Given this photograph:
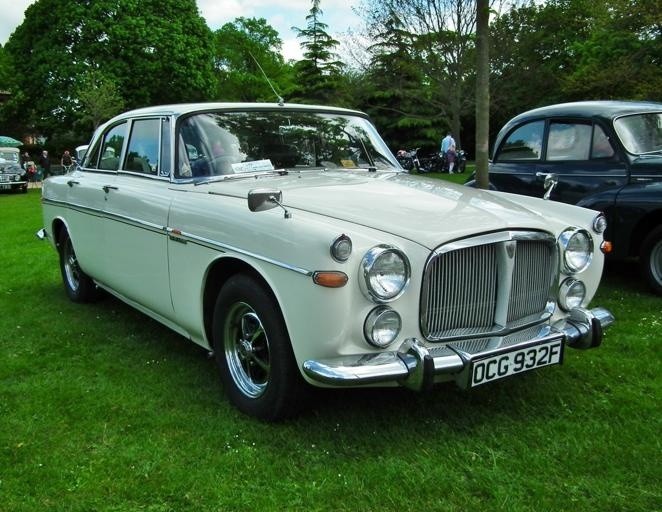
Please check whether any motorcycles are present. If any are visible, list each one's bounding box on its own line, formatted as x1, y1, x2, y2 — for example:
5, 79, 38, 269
395, 141, 467, 176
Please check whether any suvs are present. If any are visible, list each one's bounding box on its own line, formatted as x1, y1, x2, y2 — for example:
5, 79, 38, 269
0, 147, 30, 195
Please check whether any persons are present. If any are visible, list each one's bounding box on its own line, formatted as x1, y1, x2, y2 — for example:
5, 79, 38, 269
446, 144, 458, 175
60, 150, 73, 175
21, 150, 50, 180
441, 132, 456, 173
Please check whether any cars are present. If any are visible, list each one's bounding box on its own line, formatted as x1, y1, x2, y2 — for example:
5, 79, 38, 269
38, 49, 615, 423
461, 100, 662, 291
73, 144, 115, 170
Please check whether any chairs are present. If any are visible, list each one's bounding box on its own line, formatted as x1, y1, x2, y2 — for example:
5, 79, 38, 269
256, 144, 301, 169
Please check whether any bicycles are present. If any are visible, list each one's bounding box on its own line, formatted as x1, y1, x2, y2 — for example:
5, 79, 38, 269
61, 164, 74, 174
